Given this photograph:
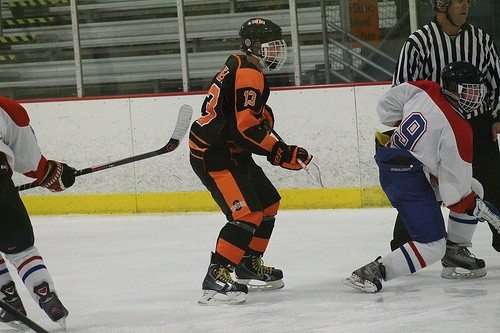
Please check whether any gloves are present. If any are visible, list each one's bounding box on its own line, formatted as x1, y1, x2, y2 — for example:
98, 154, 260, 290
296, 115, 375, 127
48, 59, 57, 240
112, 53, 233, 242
261, 105, 274, 133
467, 195, 498, 222
268, 142, 313, 171
39, 161, 76, 192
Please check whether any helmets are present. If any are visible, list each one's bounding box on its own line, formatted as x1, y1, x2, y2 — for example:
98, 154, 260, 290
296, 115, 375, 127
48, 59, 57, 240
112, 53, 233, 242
443, 62, 486, 114
240, 18, 288, 71
429, 0, 452, 11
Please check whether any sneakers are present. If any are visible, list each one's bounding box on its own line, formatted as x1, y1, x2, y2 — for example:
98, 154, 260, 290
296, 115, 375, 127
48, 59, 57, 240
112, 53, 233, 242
198, 252, 248, 305
0, 282, 29, 332
235, 255, 285, 291
441, 241, 487, 279
344, 255, 387, 292
33, 282, 70, 332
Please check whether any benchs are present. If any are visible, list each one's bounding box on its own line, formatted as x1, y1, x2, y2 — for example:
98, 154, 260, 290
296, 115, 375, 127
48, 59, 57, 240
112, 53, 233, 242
0, 0, 398, 89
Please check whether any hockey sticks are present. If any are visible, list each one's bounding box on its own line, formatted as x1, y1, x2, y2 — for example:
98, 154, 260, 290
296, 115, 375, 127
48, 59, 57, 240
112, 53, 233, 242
260, 120, 322, 187
16, 104, 193, 192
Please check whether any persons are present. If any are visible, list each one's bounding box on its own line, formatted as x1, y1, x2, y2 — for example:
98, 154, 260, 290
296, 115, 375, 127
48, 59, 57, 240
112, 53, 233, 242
353, 60, 488, 292
188, 17, 314, 294
390, 0, 500, 251
0, 95, 77, 322
